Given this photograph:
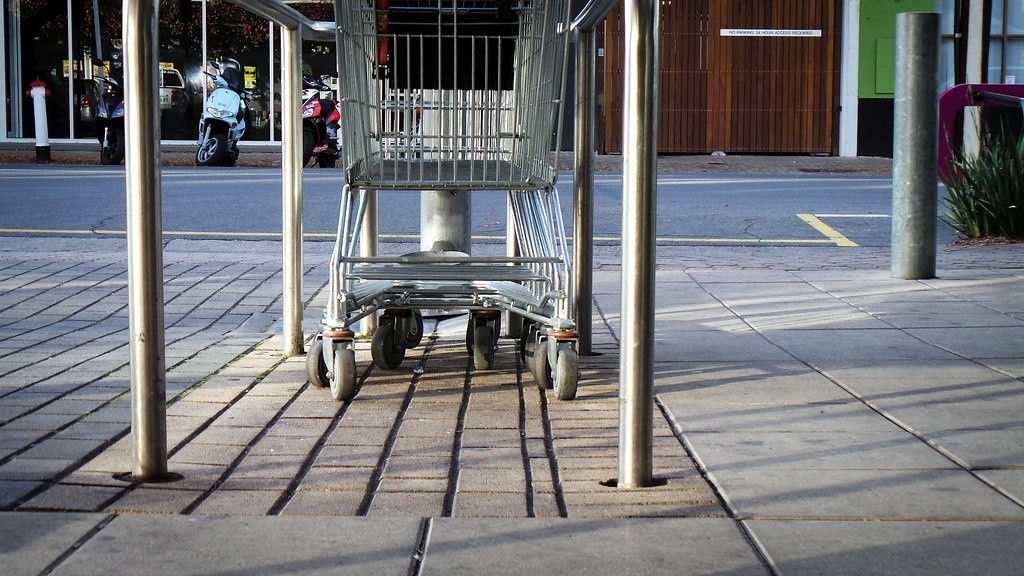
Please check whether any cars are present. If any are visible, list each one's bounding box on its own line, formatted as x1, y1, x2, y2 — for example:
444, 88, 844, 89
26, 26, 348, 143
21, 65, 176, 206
158, 67, 189, 140
67, 78, 99, 137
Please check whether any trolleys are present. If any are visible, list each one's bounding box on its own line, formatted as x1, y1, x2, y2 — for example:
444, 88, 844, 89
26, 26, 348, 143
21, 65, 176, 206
302, 1, 621, 400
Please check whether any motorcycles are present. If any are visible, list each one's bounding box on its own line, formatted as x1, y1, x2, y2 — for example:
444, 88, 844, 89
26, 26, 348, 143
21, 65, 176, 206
196, 58, 251, 166
93, 54, 124, 166
